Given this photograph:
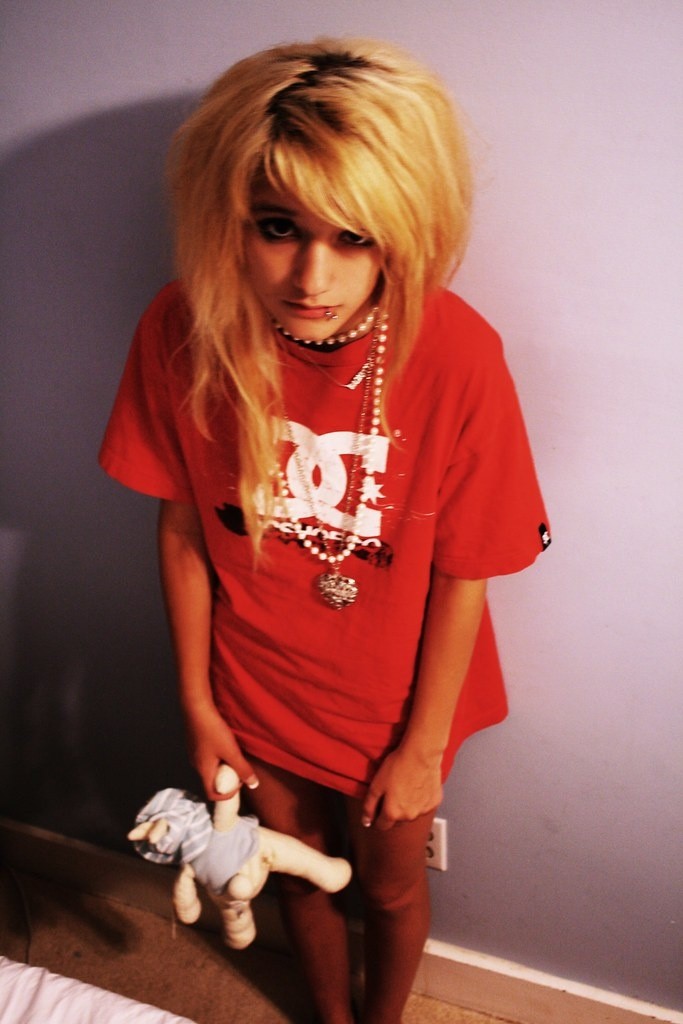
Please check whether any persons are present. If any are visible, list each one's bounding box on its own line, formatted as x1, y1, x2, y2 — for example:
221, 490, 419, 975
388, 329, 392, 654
95, 40, 549, 1023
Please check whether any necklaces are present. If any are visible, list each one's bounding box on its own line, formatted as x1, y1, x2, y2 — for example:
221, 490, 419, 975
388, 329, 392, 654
253, 308, 390, 609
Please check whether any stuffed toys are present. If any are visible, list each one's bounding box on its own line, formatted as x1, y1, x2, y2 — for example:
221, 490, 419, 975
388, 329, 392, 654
125, 764, 351, 952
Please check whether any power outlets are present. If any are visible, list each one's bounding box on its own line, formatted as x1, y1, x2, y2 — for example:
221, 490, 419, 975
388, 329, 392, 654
425, 818, 448, 872
